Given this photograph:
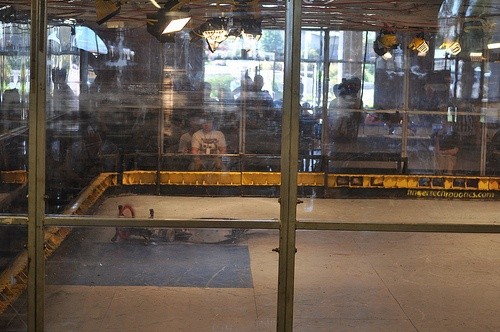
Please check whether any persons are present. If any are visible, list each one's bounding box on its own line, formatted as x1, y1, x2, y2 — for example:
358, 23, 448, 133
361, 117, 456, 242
188, 118, 231, 170
430, 103, 457, 151
52, 68, 78, 162
85, 70, 280, 172
456, 97, 477, 172
295, 76, 365, 135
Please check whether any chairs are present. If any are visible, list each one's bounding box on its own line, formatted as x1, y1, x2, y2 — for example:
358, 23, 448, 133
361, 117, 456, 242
456, 105, 478, 141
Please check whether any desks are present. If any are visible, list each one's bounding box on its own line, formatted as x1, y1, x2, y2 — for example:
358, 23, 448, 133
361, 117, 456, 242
358, 124, 432, 151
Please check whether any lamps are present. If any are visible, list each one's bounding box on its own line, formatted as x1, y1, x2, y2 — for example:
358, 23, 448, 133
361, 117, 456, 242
147, 11, 192, 33
374, 25, 398, 61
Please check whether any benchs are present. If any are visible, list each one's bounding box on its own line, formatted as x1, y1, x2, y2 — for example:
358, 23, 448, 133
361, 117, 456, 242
330, 152, 408, 174
